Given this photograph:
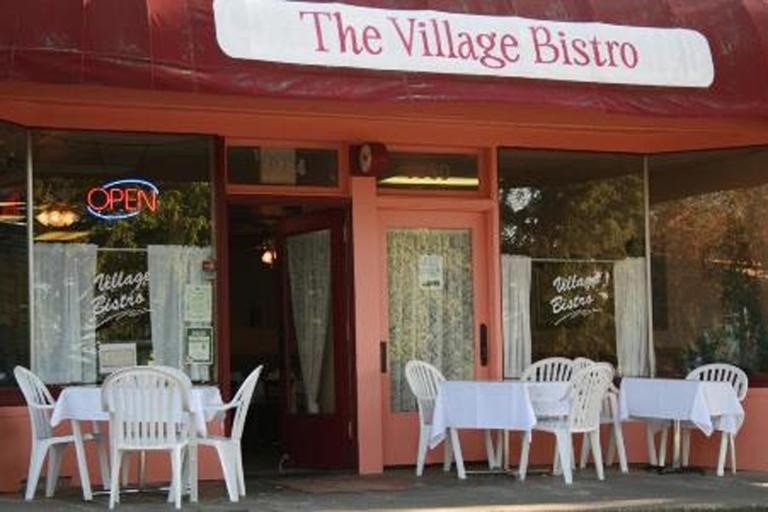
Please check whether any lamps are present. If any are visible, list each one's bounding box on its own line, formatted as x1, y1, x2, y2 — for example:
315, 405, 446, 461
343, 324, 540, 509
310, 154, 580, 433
261, 238, 277, 265
35, 200, 80, 228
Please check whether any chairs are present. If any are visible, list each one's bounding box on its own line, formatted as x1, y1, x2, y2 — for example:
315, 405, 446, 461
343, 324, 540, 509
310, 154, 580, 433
13, 363, 264, 508
406, 357, 749, 486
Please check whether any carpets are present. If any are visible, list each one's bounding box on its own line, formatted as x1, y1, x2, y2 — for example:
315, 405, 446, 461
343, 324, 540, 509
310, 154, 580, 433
283, 474, 414, 494
